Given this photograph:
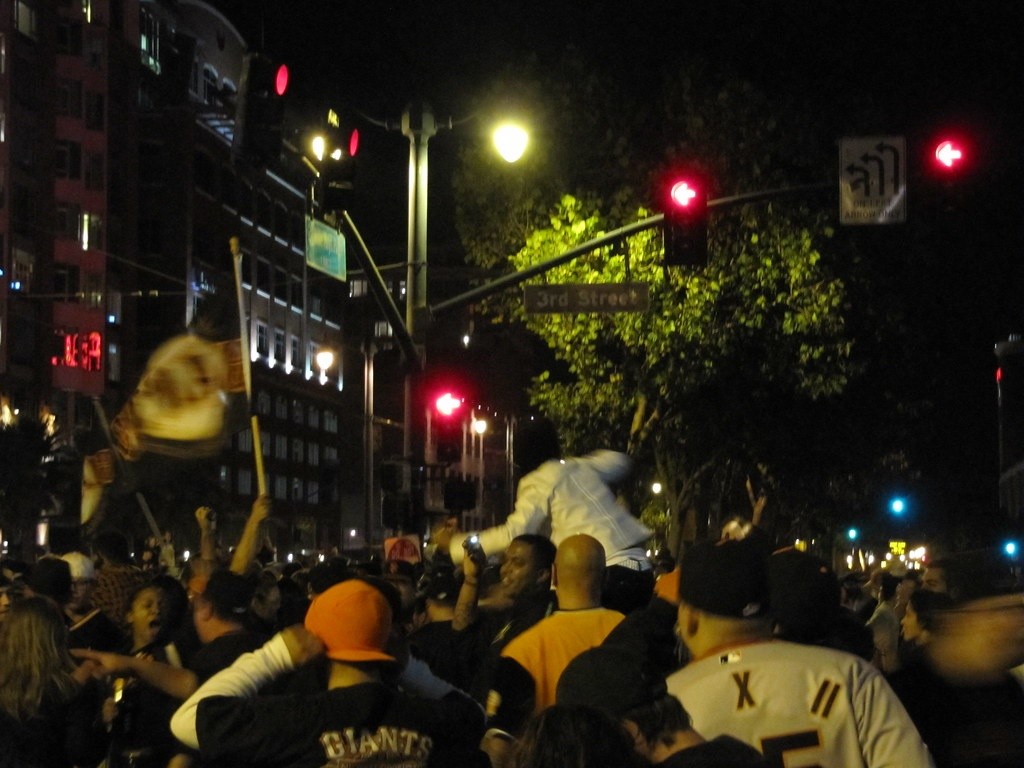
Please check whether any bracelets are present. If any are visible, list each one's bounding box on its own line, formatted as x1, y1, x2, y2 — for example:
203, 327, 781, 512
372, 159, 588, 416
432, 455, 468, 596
464, 578, 478, 588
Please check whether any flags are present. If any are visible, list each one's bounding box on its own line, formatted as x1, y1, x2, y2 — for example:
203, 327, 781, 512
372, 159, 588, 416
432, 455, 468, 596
76, 271, 248, 538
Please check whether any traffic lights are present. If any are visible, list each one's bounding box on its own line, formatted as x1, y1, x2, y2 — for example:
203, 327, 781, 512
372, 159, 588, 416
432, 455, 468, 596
660, 166, 708, 266
298, 103, 362, 225
422, 318, 467, 424
928, 118, 977, 199
230, 53, 296, 164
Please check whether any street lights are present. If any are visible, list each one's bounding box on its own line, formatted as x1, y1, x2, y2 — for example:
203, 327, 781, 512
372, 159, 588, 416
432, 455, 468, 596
304, 92, 538, 570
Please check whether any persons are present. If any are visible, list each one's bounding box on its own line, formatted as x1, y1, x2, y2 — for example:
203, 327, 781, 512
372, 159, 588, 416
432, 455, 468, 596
0, 441, 1024, 768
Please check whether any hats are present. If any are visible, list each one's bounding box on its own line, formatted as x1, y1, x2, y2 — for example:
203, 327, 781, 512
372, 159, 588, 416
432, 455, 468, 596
304, 580, 395, 661
61, 551, 95, 580
678, 543, 772, 618
384, 560, 415, 582
